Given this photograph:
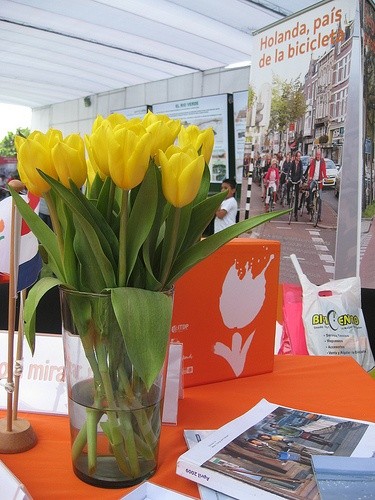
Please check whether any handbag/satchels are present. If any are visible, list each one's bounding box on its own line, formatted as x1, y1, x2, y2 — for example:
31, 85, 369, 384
302, 276, 375, 373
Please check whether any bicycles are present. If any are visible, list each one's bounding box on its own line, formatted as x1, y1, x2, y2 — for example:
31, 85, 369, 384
242, 156, 311, 210
267, 179, 278, 211
309, 179, 324, 228
286, 177, 304, 225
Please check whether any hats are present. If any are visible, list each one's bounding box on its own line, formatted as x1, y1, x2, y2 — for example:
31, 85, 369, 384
270, 159, 277, 164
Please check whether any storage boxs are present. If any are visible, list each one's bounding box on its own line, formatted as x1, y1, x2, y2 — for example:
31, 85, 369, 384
163, 238, 281, 384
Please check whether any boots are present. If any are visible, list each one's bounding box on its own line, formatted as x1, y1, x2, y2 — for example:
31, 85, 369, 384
264, 203, 269, 213
273, 192, 276, 204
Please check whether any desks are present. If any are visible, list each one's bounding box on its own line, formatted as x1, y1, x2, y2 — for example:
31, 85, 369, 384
0, 354, 375, 500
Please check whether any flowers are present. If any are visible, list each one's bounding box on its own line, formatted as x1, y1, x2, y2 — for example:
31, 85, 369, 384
5, 108, 294, 475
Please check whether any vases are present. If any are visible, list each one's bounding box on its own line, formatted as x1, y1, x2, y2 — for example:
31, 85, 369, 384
58, 284, 176, 489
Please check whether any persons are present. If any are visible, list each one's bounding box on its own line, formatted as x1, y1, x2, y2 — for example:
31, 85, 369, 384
242, 147, 328, 222
214, 178, 238, 234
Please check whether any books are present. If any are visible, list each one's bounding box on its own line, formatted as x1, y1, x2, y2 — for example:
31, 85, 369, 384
176, 398, 375, 500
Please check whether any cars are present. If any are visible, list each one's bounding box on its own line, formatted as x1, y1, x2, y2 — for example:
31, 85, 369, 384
335, 166, 341, 197
322, 158, 338, 190
299, 156, 310, 176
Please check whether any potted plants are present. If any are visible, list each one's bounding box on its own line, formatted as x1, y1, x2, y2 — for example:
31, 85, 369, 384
0, 126, 31, 189
